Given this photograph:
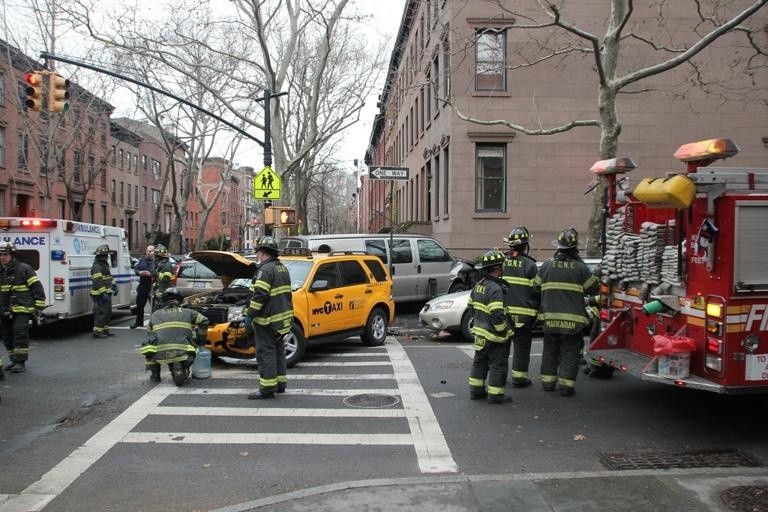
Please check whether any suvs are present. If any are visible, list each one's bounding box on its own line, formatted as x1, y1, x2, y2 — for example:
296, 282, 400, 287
177, 249, 397, 370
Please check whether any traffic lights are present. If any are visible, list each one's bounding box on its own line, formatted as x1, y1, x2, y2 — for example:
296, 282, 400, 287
279, 208, 298, 226
23, 72, 43, 111
47, 74, 69, 113
263, 208, 276, 226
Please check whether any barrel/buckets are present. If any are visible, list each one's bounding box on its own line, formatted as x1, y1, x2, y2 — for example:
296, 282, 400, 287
192, 347, 212, 378
656, 349, 690, 380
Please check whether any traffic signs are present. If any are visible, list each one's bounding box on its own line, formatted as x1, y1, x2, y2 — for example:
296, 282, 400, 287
368, 164, 410, 181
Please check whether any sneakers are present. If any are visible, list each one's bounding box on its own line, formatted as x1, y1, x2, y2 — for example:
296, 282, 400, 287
3, 359, 27, 373
246, 387, 286, 400
92, 320, 144, 339
469, 378, 577, 405
171, 361, 187, 388
148, 371, 162, 382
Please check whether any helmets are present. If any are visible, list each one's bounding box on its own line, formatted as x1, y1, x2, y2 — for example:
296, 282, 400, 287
474, 250, 506, 271
0, 240, 18, 254
551, 227, 579, 250
91, 244, 111, 256
152, 244, 172, 259
500, 225, 530, 247
253, 236, 280, 252
158, 286, 186, 299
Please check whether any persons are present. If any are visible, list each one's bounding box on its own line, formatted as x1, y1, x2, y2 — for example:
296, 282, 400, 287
150, 244, 175, 312
498, 224, 539, 387
140, 286, 211, 385
0, 240, 47, 373
89, 244, 119, 338
533, 227, 599, 396
244, 235, 294, 400
130, 245, 155, 329
468, 246, 514, 405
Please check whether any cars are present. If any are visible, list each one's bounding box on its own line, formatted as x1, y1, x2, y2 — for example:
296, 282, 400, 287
417, 257, 602, 343
162, 252, 263, 300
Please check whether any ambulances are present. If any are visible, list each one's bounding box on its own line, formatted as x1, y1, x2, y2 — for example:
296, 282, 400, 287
0, 215, 142, 338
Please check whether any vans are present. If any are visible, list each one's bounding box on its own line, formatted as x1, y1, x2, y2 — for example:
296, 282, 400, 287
278, 231, 482, 304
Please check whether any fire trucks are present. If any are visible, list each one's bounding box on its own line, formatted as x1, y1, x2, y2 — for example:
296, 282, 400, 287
584, 136, 767, 400
